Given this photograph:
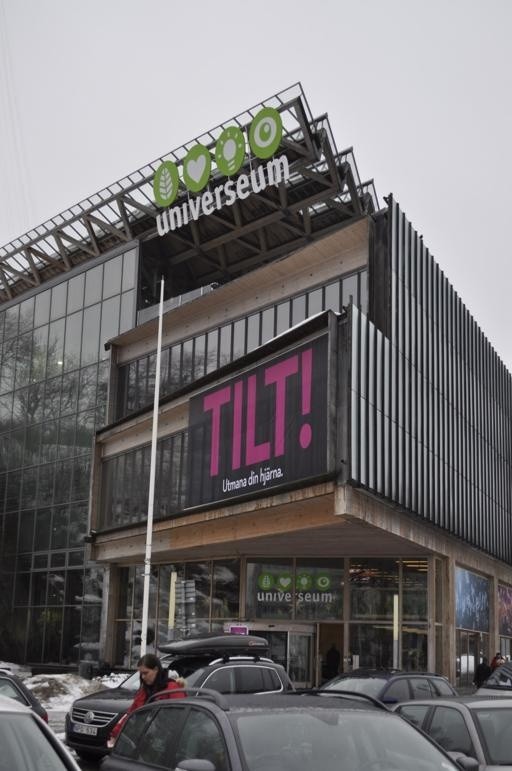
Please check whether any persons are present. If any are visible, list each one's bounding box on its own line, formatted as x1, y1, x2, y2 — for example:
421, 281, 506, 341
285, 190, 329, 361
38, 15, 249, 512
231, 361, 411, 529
475, 653, 489, 681
324, 641, 341, 677
492, 652, 506, 672
129, 651, 188, 714
